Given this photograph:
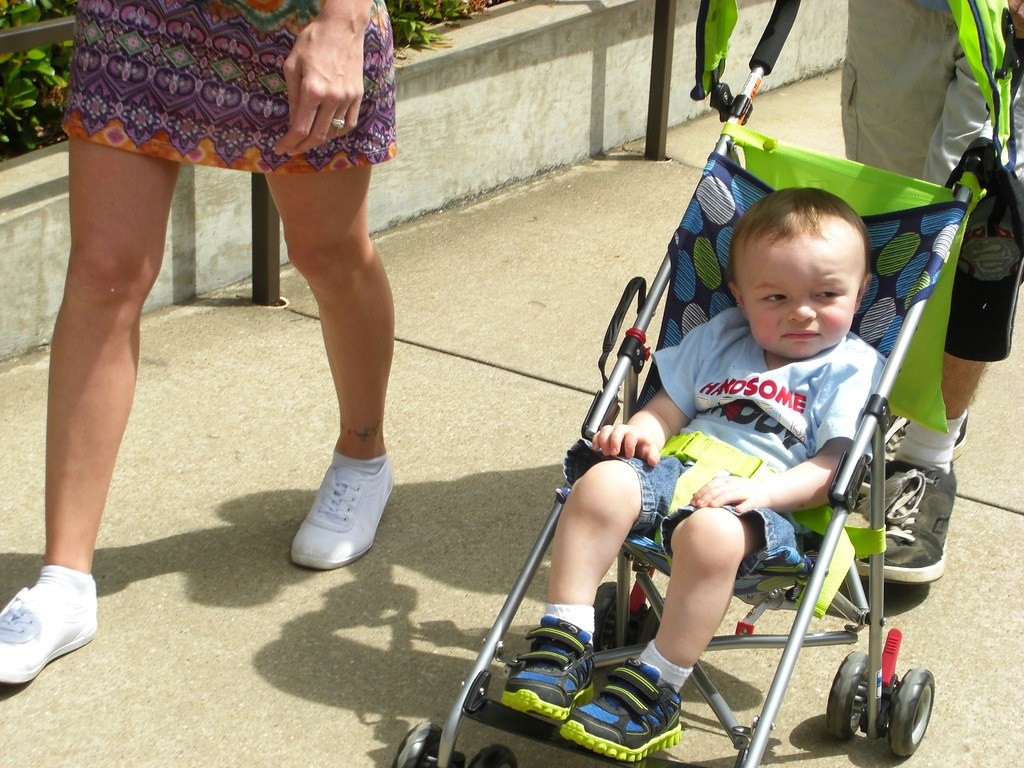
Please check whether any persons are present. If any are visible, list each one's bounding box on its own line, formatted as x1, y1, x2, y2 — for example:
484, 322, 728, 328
502, 188, 886, 761
0, 0, 396, 683
841, 1, 1024, 583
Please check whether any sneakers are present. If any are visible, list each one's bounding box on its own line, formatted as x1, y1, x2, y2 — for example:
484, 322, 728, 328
290, 455, 394, 570
856, 413, 969, 496
0, 579, 98, 684
500, 614, 595, 725
559, 657, 682, 762
855, 462, 957, 586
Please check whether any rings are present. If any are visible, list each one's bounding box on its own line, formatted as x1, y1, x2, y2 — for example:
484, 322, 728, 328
332, 119, 344, 128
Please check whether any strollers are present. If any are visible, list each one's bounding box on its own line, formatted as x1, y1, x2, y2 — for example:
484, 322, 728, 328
389, 0, 1024, 768
